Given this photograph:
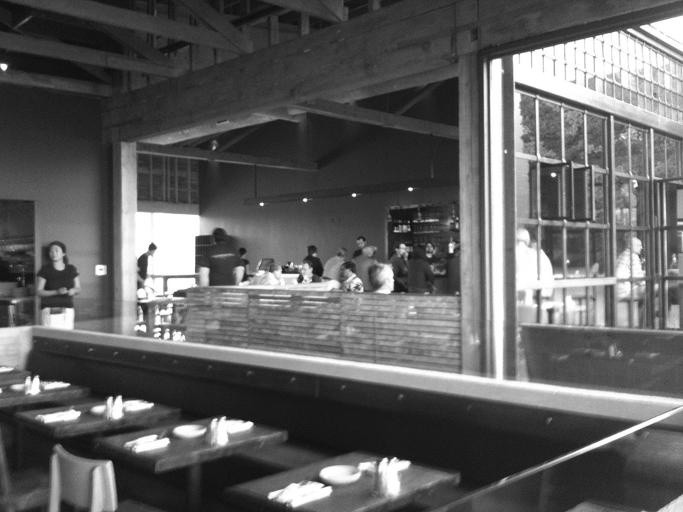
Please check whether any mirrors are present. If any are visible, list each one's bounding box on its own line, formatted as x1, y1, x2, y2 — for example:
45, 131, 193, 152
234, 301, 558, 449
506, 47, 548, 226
1, 199, 41, 328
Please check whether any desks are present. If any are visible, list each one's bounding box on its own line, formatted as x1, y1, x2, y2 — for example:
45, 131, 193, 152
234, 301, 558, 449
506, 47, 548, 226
245, 272, 300, 285
136, 274, 198, 341
0, 365, 461, 512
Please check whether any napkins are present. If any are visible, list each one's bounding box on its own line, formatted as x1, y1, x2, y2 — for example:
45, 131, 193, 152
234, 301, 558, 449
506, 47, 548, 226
357, 458, 412, 475
41, 381, 70, 391
377, 457, 402, 499
123, 400, 154, 413
1, 366, 15, 373
33, 407, 81, 423
267, 479, 333, 508
24, 375, 42, 395
122, 434, 171, 453
207, 415, 229, 447
10, 383, 24, 390
102, 395, 124, 421
227, 420, 254, 434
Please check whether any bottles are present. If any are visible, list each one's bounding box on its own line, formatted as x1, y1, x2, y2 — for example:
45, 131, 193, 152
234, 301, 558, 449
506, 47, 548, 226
374, 456, 402, 497
392, 219, 412, 232
103, 395, 123, 420
208, 416, 228, 445
23, 374, 40, 395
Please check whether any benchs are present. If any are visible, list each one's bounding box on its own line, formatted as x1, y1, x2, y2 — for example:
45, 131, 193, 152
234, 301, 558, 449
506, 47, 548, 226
23, 328, 683, 512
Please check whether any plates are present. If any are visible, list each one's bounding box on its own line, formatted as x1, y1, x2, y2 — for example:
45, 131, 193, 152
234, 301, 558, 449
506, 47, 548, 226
318, 464, 361, 486
172, 424, 206, 439
10, 383, 23, 391
0, 367, 13, 373
90, 405, 105, 414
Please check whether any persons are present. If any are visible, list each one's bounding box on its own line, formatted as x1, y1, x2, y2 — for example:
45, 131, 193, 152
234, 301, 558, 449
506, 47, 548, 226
303, 235, 460, 295
199, 228, 244, 287
531, 240, 554, 302
238, 248, 254, 281
616, 237, 659, 300
137, 242, 157, 288
36, 240, 81, 324
514, 229, 537, 300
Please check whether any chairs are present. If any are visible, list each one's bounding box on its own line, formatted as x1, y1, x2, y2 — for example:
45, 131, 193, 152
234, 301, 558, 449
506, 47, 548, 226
0, 436, 66, 511
47, 443, 162, 511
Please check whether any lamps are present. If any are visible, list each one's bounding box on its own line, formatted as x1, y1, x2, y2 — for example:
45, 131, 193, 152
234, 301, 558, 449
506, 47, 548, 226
210, 139, 218, 152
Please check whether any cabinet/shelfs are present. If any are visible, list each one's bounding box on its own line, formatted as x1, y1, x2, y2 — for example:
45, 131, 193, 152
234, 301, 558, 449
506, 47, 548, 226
385, 201, 459, 295
195, 235, 215, 285
